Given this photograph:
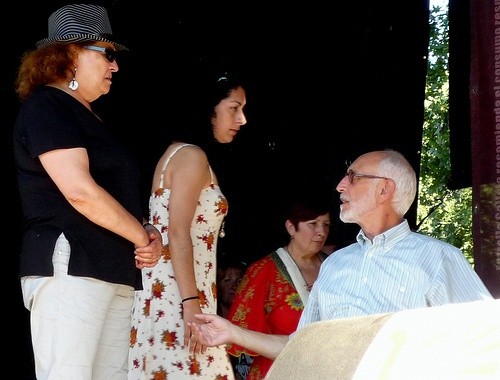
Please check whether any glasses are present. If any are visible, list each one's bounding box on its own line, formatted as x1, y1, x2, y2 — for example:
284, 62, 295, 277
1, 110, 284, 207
83, 45, 122, 62
345, 169, 384, 184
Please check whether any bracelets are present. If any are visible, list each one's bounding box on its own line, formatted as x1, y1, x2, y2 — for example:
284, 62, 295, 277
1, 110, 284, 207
142, 223, 151, 227
182, 296, 200, 302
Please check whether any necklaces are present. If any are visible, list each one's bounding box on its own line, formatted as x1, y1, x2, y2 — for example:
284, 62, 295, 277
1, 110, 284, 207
286, 247, 323, 293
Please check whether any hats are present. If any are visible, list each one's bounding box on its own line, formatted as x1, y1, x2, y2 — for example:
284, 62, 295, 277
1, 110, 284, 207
36, 4, 130, 54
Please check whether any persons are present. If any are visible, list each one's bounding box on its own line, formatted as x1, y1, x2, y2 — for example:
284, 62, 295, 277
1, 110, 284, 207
12, 4, 163, 380
226, 194, 330, 380
128, 71, 247, 380
217, 261, 255, 380
187, 149, 495, 361
